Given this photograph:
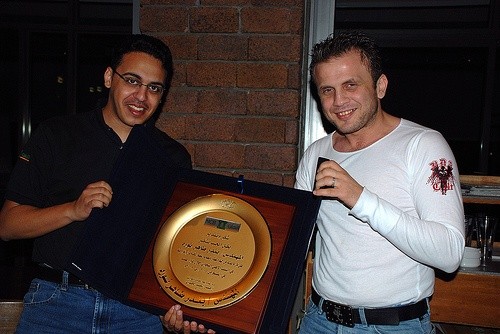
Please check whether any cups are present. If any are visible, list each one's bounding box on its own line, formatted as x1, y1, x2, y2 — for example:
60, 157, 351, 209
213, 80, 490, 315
477, 215, 494, 267
464, 215, 473, 246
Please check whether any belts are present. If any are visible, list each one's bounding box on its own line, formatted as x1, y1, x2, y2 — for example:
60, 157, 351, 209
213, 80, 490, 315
310, 284, 433, 328
32, 264, 87, 286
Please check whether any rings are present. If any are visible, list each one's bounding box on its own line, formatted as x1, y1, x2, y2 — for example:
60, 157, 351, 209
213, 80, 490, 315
331, 177, 336, 186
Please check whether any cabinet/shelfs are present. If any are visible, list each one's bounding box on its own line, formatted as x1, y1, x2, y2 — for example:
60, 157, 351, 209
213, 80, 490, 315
305, 174, 500, 330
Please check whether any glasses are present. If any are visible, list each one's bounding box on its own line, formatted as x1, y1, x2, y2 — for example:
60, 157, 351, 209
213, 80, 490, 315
112, 69, 167, 94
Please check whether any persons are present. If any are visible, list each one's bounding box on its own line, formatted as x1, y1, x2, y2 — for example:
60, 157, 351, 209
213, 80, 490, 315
184, 34, 466, 334
0, 33, 185, 334
